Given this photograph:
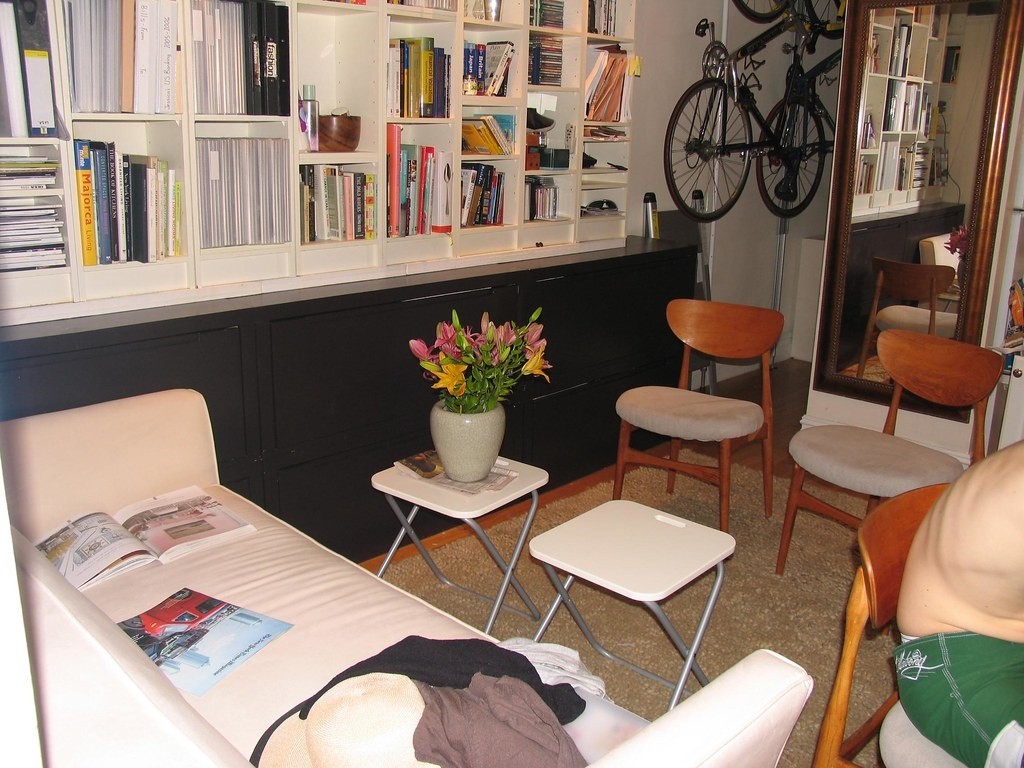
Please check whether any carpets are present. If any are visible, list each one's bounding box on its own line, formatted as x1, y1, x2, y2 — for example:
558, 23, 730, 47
379, 448, 901, 768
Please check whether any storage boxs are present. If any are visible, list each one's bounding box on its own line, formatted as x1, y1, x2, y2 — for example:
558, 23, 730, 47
539, 148, 569, 169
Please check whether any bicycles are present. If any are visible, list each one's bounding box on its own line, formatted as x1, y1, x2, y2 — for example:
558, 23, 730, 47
663, 0, 847, 219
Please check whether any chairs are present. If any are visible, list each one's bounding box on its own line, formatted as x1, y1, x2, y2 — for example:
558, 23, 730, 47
614, 299, 784, 532
813, 483, 970, 768
856, 256, 958, 384
642, 190, 716, 395
774, 328, 1005, 574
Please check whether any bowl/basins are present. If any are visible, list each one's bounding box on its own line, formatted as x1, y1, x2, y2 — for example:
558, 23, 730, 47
319, 115, 361, 152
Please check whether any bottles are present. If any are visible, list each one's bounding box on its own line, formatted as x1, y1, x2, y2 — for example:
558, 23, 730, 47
301, 86, 319, 152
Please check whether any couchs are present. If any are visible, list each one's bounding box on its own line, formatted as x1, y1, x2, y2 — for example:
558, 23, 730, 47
0, 388, 814, 768
918, 232, 960, 314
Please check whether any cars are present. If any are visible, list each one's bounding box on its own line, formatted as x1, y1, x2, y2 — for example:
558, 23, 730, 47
122, 588, 228, 641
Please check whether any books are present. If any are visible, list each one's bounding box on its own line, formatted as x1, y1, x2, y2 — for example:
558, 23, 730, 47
856, 18, 949, 195
588, 0, 617, 37
63, 0, 290, 116
387, 35, 452, 118
0, 1, 58, 137
461, 163, 505, 227
462, 112, 516, 156
32, 485, 258, 593
525, 177, 558, 221
387, 124, 454, 238
585, 44, 631, 139
0, 155, 66, 271
196, 137, 291, 249
324, 0, 367, 5
528, 0, 564, 87
74, 139, 182, 266
463, 39, 515, 98
300, 165, 378, 245
388, 0, 457, 11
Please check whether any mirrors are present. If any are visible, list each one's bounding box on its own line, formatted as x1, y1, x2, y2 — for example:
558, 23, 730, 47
811, 0, 1024, 424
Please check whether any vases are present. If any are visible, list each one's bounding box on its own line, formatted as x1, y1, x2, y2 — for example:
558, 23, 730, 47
430, 398, 505, 482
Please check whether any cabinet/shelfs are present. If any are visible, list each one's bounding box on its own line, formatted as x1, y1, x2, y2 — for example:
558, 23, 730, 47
0, 0, 698, 565
841, 3, 968, 328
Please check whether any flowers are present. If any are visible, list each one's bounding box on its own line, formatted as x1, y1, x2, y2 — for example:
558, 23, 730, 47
408, 307, 552, 416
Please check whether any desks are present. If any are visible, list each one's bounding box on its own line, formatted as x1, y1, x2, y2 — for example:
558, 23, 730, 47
372, 453, 549, 633
528, 499, 736, 713
